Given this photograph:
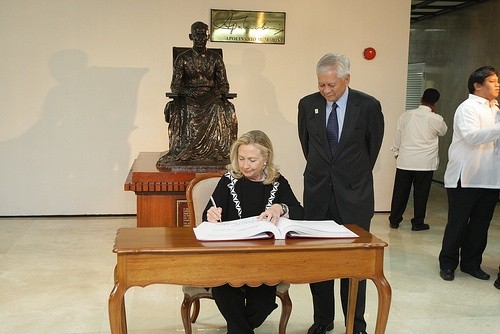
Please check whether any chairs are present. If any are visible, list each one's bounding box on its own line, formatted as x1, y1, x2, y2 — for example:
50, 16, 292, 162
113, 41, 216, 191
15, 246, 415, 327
181, 172, 292, 334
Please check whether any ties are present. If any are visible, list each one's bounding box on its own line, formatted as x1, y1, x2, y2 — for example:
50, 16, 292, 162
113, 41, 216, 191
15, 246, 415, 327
326, 102, 338, 159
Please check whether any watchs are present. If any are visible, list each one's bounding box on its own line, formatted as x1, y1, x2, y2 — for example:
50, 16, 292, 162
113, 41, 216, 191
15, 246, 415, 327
280, 203, 288, 217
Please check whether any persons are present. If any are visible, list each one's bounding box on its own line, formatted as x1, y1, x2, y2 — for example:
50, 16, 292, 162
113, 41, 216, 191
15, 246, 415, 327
389, 88, 448, 231
440, 66, 500, 281
159, 21, 238, 163
201, 131, 305, 334
298, 53, 385, 334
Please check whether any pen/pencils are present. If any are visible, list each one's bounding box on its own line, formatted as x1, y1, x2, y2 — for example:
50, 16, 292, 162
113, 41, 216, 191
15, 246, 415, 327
209, 195, 222, 222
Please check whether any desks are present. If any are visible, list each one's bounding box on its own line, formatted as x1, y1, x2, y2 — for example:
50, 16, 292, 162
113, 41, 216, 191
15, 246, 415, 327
109, 223, 392, 334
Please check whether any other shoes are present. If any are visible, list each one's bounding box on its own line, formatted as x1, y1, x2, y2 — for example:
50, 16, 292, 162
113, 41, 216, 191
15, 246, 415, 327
412, 224, 429, 230
390, 221, 399, 228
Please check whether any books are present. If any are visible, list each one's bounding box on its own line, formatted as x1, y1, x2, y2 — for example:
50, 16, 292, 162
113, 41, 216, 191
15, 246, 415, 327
193, 215, 360, 243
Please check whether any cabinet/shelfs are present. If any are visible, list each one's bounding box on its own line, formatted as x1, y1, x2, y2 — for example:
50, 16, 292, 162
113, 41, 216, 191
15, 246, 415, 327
124, 152, 228, 228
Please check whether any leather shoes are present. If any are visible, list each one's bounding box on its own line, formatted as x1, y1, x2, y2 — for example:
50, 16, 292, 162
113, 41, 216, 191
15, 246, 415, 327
308, 323, 334, 334
354, 331, 367, 334
461, 266, 490, 280
440, 266, 454, 280
